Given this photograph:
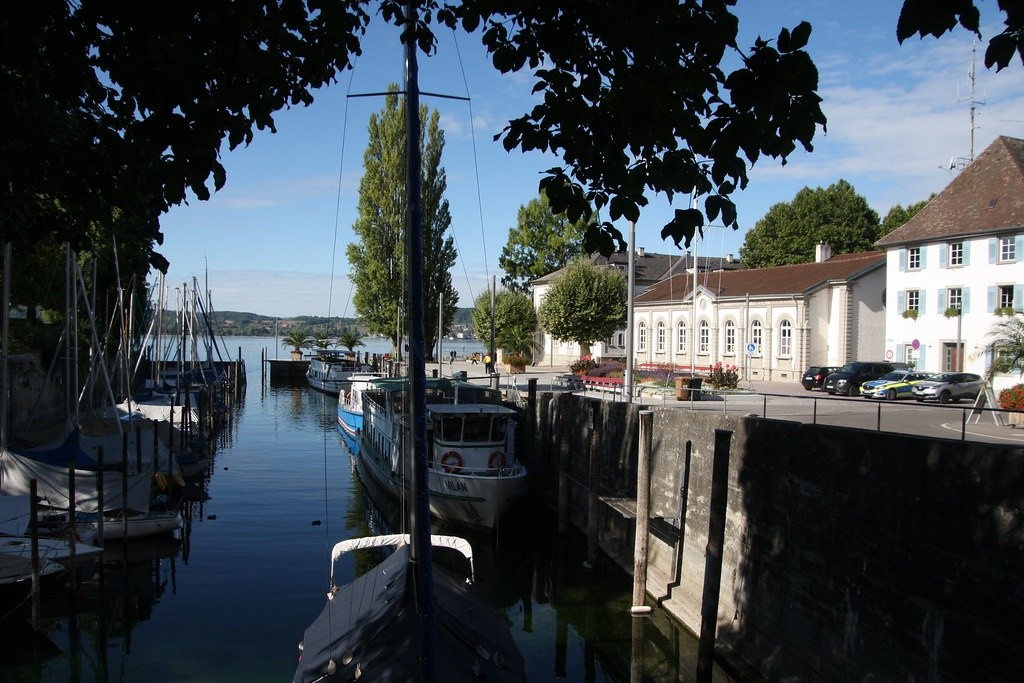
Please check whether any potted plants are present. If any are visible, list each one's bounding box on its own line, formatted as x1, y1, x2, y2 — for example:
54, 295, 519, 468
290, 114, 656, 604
312, 332, 334, 360
281, 332, 314, 361
336, 331, 368, 361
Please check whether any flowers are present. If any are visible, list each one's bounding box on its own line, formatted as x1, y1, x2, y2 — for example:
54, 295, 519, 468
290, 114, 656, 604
503, 357, 531, 366
999, 383, 1024, 411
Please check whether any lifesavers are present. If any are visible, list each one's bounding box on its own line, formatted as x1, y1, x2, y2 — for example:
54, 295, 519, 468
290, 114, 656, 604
441, 451, 463, 474
487, 451, 506, 469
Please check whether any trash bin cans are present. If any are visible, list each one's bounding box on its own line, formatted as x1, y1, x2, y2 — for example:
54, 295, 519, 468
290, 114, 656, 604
492, 372, 500, 387
673, 377, 703, 401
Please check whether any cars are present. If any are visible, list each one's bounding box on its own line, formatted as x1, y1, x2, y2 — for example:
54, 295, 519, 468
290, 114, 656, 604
858, 366, 935, 400
911, 371, 984, 404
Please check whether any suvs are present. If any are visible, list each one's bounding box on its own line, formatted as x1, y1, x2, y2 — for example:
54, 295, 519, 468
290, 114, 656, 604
802, 365, 841, 391
822, 361, 910, 397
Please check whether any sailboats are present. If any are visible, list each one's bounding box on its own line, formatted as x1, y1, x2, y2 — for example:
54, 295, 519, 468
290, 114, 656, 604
262, 2, 532, 683
0, 229, 248, 611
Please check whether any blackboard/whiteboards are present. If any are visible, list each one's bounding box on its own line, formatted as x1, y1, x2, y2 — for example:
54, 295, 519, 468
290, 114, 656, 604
970, 386, 987, 415
984, 385, 1001, 414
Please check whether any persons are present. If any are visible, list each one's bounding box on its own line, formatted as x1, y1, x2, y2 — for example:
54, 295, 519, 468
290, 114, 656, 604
449, 350, 457, 364
481, 351, 497, 374
471, 353, 478, 365
